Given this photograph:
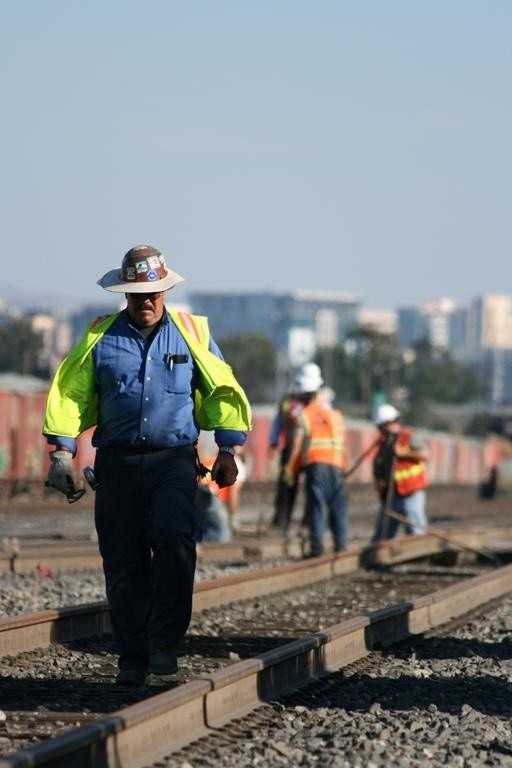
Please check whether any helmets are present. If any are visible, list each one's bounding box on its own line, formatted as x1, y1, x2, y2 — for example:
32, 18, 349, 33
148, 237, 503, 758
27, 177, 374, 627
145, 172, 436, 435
375, 404, 400, 425
97, 244, 185, 293
287, 361, 324, 395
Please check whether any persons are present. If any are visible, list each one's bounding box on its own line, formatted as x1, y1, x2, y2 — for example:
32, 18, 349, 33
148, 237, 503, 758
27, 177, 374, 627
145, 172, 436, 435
42, 246, 253, 694
196, 444, 247, 543
266, 362, 430, 558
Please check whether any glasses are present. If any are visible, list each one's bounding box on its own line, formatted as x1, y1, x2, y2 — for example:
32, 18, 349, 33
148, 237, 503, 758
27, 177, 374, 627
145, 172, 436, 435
127, 292, 160, 300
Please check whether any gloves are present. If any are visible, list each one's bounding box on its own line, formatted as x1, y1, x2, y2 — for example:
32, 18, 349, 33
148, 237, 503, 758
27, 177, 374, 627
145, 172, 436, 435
45, 450, 86, 504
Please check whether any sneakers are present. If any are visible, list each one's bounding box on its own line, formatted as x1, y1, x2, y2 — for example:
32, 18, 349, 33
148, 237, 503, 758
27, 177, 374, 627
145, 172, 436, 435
116, 649, 178, 686
304, 544, 346, 558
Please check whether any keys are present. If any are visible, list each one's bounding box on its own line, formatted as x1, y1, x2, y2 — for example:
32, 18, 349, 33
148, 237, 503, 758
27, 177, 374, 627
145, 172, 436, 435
197, 463, 211, 482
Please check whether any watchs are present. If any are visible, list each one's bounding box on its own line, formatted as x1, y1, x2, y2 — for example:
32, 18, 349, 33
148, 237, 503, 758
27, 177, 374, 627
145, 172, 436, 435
219, 447, 235, 456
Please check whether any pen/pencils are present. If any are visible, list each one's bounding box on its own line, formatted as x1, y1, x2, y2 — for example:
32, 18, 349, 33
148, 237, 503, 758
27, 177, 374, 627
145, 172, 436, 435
170, 357, 173, 371
